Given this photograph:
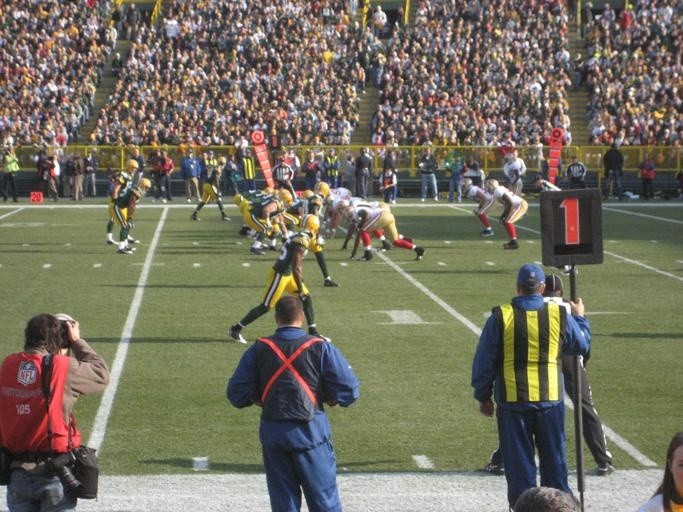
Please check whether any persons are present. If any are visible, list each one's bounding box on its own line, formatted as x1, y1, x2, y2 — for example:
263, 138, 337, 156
0, 313, 109, 512
634, 433, 683, 512
233, 183, 426, 262
111, 177, 151, 255
471, 266, 592, 510
105, 159, 141, 245
227, 214, 331, 346
0, 0, 683, 201
511, 485, 581, 511
302, 182, 340, 288
226, 295, 358, 512
459, 177, 495, 235
486, 179, 528, 250
482, 274, 616, 475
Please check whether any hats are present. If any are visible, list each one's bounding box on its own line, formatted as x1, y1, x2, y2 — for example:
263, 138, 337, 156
529, 175, 541, 184
518, 265, 546, 292
546, 274, 563, 292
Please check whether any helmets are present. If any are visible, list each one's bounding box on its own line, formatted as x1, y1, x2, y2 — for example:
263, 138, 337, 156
126, 159, 138, 170
218, 157, 226, 167
485, 179, 499, 196
139, 178, 151, 188
264, 181, 356, 223
459, 178, 473, 193
107, 239, 140, 254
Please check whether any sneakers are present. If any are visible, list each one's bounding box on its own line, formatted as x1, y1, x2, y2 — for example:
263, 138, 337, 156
480, 229, 519, 249
313, 333, 332, 344
191, 213, 200, 221
597, 463, 614, 475
222, 216, 231, 221
325, 279, 340, 286
239, 214, 424, 261
484, 463, 505, 475
227, 325, 248, 344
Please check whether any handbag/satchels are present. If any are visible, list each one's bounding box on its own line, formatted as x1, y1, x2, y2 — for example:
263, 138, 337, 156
70, 444, 98, 498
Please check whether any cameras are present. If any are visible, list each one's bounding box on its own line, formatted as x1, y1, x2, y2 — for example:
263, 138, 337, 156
55, 312, 79, 353
